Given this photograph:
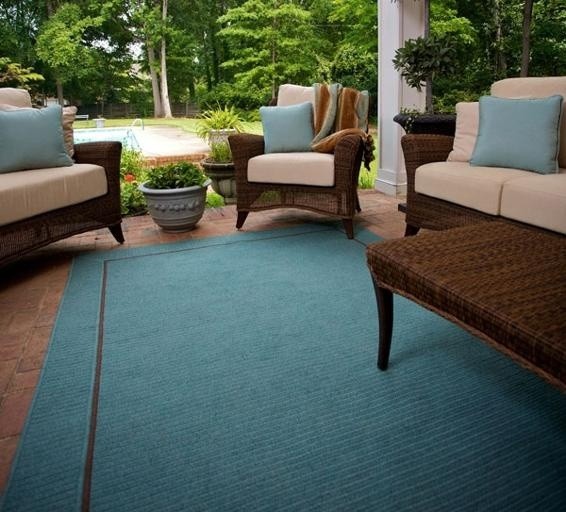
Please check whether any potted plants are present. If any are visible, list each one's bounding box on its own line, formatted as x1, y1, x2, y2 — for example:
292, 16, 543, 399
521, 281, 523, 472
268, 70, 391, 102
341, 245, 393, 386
392, 34, 470, 133
138, 161, 212, 234
192, 97, 254, 205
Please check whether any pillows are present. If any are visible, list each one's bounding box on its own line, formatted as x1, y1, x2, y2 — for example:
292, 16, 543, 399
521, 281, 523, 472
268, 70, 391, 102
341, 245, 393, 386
0, 103, 78, 173
446, 95, 563, 174
260, 102, 315, 153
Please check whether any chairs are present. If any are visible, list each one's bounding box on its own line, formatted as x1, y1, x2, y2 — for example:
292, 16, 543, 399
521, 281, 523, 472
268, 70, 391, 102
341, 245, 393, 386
227, 83, 370, 239
401, 75, 566, 236
0, 86, 124, 264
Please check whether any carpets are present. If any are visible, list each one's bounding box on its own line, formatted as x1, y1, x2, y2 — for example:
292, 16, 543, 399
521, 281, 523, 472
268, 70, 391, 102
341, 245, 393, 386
0, 219, 566, 512
